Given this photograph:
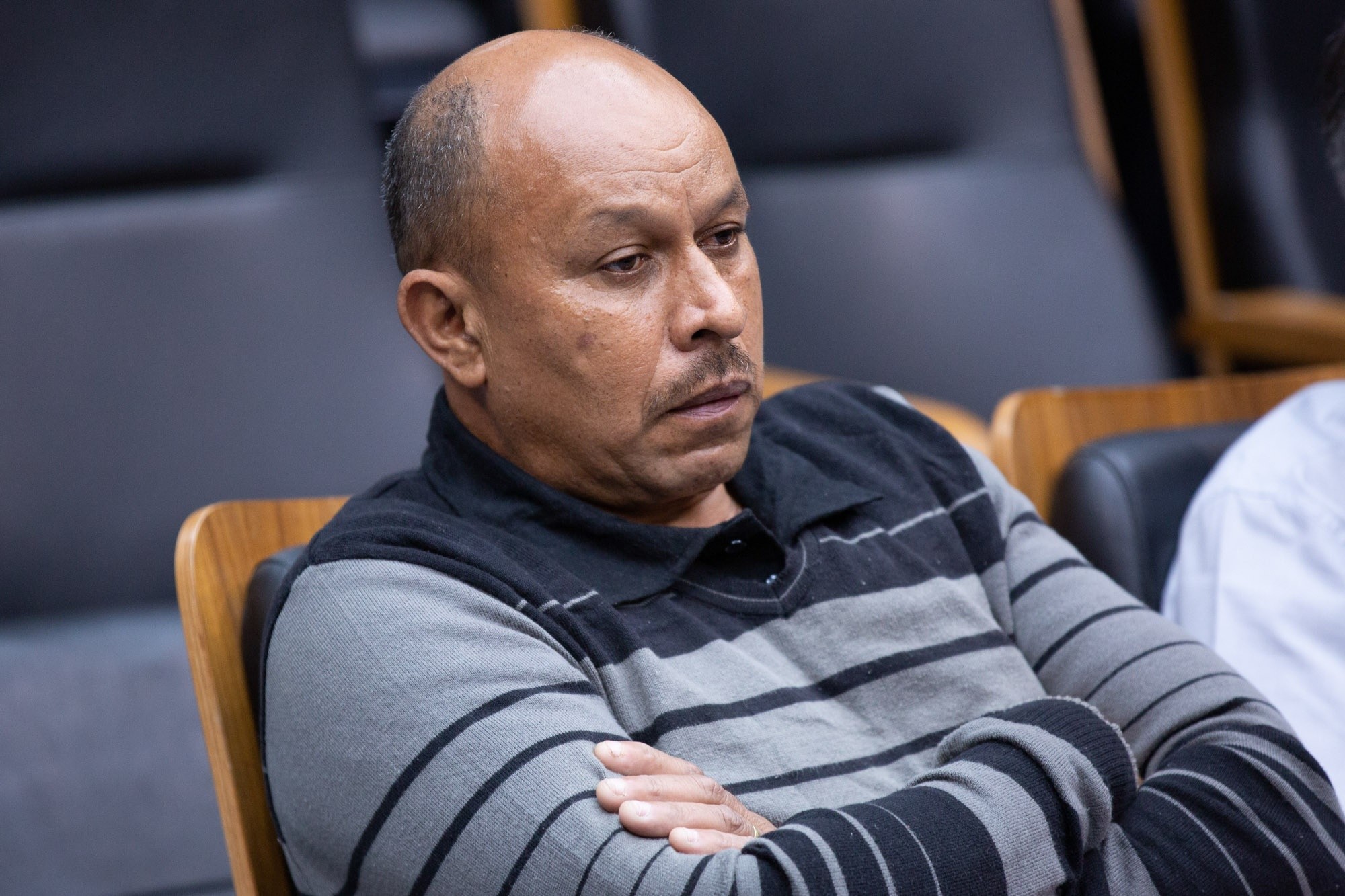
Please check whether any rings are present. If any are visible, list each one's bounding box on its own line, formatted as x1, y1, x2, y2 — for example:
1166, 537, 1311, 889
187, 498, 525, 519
753, 825, 759, 838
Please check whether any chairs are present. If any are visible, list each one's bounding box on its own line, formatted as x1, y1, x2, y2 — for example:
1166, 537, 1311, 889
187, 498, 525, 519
0, 0, 1344, 895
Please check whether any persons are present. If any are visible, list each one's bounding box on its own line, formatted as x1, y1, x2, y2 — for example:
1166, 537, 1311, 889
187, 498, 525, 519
1158, 376, 1345, 814
240, 23, 1345, 896
